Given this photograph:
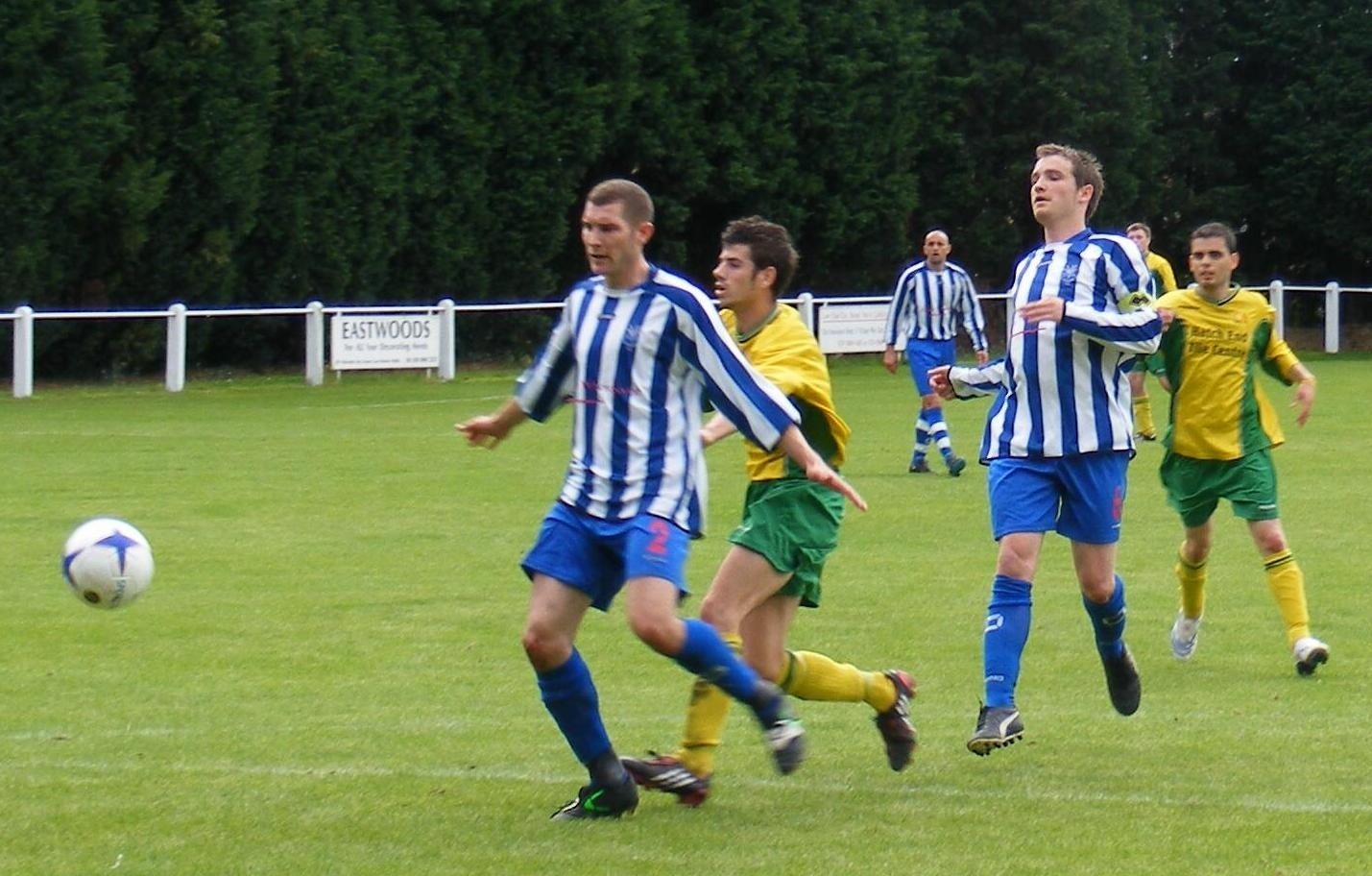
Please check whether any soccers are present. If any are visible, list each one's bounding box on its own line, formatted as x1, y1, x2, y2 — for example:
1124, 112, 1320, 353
62, 517, 158, 611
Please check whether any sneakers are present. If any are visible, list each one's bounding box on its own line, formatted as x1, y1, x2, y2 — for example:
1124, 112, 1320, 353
549, 770, 639, 822
1171, 608, 1203, 658
948, 456, 967, 474
910, 461, 936, 473
619, 749, 713, 809
1134, 433, 1157, 444
758, 685, 804, 775
965, 692, 1026, 756
1101, 637, 1142, 717
1292, 636, 1331, 675
869, 668, 916, 771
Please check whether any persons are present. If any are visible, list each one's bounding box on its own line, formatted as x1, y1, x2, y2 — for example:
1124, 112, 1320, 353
880, 229, 990, 479
448, 177, 872, 822
1151, 222, 1330, 676
613, 216, 919, 803
925, 142, 1165, 759
1124, 221, 1180, 442
